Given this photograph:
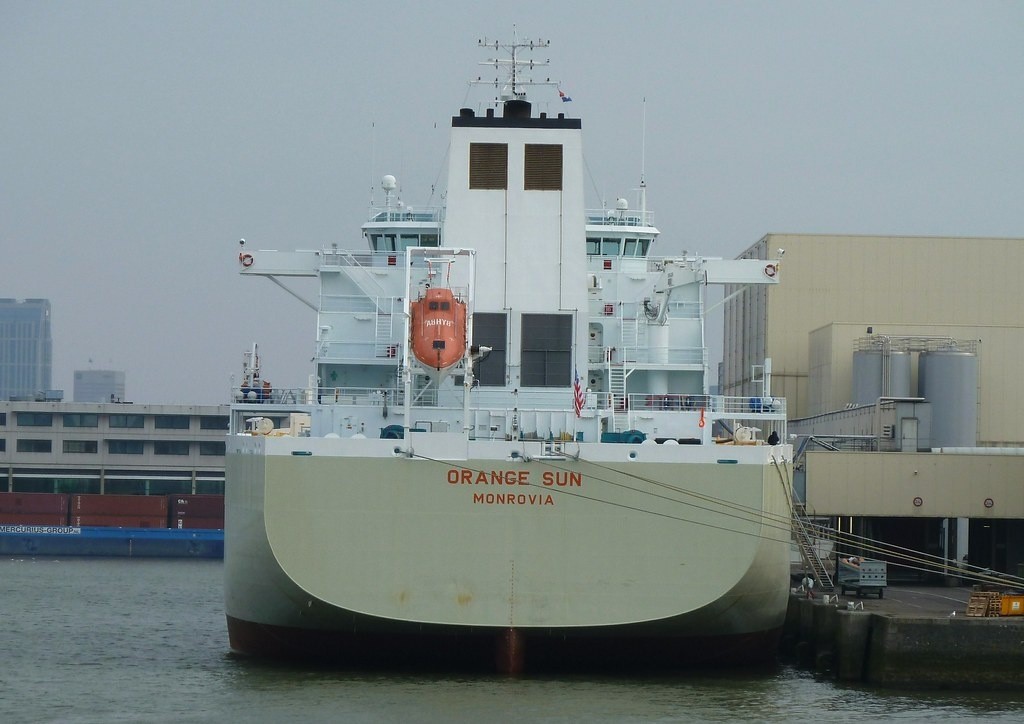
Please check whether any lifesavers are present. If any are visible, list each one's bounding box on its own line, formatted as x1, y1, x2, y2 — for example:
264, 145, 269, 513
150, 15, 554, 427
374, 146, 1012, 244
765, 264, 776, 276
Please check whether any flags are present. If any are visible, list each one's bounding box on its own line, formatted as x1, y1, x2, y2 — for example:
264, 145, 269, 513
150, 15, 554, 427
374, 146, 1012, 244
574, 370, 585, 418
560, 91, 572, 102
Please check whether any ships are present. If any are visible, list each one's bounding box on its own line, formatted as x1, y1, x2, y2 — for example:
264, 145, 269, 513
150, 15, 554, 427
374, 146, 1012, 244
223, 26, 796, 681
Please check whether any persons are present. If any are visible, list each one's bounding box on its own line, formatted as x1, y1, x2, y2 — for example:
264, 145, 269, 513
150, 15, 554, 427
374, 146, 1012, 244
768, 431, 779, 445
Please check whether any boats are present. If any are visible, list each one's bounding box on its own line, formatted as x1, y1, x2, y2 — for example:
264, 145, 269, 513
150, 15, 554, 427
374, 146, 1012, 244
239, 380, 270, 399
410, 285, 467, 387
